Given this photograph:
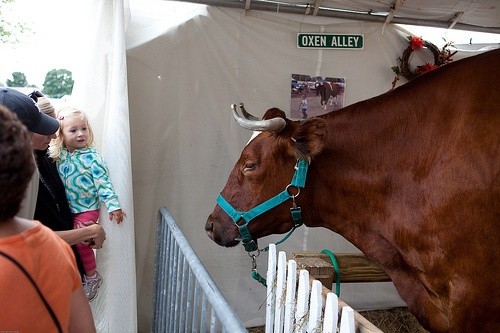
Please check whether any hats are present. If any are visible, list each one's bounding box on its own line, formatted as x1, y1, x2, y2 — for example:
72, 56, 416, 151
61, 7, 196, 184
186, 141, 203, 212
0, 88, 60, 135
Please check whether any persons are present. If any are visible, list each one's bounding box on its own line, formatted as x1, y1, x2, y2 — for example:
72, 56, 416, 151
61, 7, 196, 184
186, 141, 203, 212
299, 94, 309, 119
46, 105, 127, 303
0, 88, 106, 285
0, 103, 96, 333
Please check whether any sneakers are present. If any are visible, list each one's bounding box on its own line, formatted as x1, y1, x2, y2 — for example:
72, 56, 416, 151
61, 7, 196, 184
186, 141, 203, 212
82, 270, 103, 302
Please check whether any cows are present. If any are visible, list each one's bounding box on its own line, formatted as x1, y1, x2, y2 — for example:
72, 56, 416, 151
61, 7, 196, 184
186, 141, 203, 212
314, 81, 341, 110
204, 46, 500, 333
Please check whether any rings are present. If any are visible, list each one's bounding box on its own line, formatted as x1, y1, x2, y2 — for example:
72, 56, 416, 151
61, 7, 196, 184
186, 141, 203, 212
92, 239, 94, 241
104, 237, 106, 240
99, 245, 103, 248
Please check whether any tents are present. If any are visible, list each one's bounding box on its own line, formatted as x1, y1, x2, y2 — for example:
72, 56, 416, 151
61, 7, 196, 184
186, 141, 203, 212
74, 1, 500, 333
